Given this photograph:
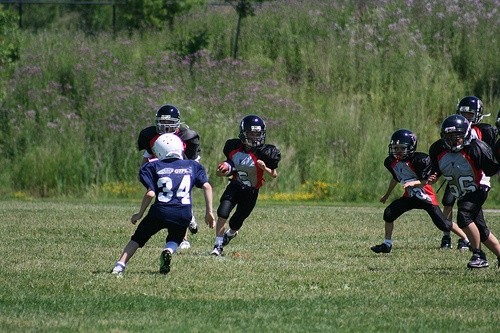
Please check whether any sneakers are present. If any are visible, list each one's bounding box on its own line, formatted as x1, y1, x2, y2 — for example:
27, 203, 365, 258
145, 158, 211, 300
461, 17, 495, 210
224, 229, 238, 246
211, 243, 223, 255
440, 235, 452, 249
371, 243, 392, 253
159, 248, 173, 274
457, 239, 467, 249
189, 217, 198, 234
109, 262, 125, 274
466, 242, 473, 251
180, 239, 190, 249
467, 249, 489, 268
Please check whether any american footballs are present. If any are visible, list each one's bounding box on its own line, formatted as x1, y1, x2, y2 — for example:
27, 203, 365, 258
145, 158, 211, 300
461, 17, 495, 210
219, 160, 237, 177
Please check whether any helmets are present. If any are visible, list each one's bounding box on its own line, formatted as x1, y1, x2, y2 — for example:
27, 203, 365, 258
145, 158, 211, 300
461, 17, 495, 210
238, 115, 266, 148
388, 129, 417, 160
154, 133, 183, 161
456, 96, 484, 125
440, 115, 471, 152
154, 105, 181, 134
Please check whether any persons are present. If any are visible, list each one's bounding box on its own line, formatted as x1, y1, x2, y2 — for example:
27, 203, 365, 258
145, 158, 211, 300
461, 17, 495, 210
425, 96, 500, 269
110, 132, 216, 274
370, 128, 472, 254
137, 104, 201, 251
210, 115, 281, 256
